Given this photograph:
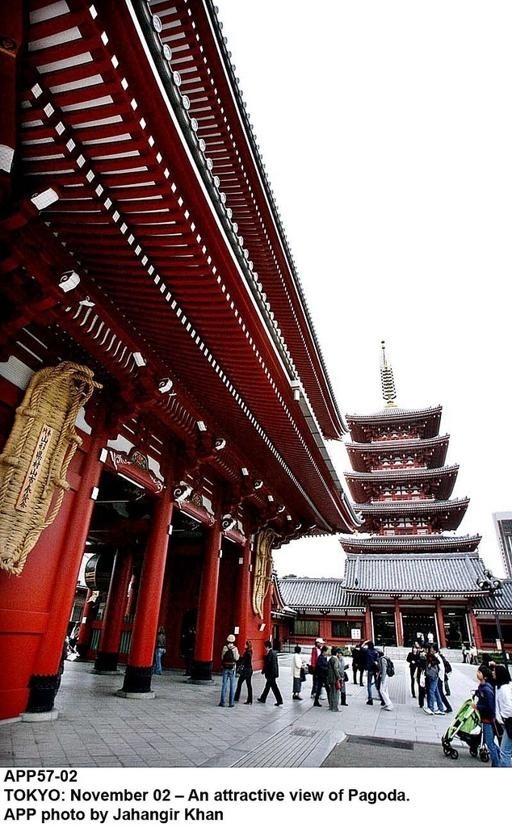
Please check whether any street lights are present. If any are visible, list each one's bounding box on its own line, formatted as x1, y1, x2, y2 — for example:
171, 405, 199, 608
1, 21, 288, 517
475, 568, 512, 682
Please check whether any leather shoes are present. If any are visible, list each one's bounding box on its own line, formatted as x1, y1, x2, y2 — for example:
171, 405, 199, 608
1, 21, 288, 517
274, 701, 283, 706
257, 698, 265, 703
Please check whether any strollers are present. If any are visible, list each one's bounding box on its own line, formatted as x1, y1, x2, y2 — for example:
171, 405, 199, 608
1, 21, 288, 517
441, 691, 491, 763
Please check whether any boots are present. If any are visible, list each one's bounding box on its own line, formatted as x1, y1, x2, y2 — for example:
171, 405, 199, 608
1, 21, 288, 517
340, 692, 348, 706
313, 696, 321, 707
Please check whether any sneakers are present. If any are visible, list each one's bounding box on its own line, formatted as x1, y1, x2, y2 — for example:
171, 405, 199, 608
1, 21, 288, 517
218, 703, 224, 707
412, 692, 452, 715
354, 681, 364, 686
381, 703, 393, 711
380, 700, 385, 706
228, 703, 234, 707
366, 698, 373, 706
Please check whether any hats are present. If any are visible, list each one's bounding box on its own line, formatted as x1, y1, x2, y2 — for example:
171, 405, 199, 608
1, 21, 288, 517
316, 637, 326, 644
227, 634, 236, 643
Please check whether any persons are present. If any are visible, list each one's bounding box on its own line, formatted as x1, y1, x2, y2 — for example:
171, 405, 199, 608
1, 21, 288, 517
60, 633, 68, 674
69, 621, 81, 653
154, 626, 511, 767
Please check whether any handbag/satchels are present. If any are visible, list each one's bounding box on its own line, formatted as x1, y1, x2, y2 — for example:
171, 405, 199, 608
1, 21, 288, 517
342, 671, 348, 681
492, 714, 504, 739
336, 678, 343, 689
236, 660, 245, 675
298, 667, 306, 681
443, 659, 451, 673
307, 663, 313, 674
502, 714, 512, 741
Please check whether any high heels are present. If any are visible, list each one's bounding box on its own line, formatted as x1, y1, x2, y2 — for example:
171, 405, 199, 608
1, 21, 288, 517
293, 694, 303, 700
233, 697, 240, 701
243, 699, 252, 705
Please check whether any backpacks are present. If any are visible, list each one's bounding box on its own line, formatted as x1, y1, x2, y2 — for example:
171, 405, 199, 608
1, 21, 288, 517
353, 659, 362, 670
384, 657, 395, 677
222, 646, 236, 670
